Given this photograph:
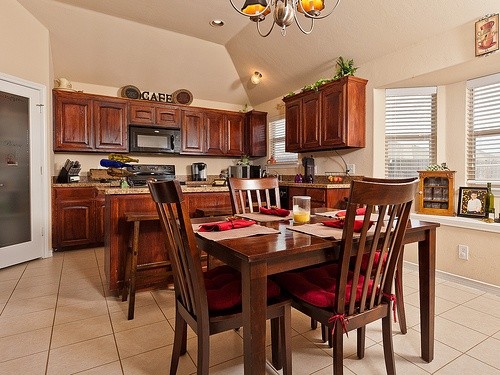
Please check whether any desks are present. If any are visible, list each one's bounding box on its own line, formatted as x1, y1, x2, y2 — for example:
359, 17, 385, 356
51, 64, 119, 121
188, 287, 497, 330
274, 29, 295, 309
190, 208, 441, 375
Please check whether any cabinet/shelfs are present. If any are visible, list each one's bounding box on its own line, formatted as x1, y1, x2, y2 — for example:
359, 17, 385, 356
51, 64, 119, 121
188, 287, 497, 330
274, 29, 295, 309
282, 76, 368, 210
53, 188, 246, 252
52, 89, 268, 158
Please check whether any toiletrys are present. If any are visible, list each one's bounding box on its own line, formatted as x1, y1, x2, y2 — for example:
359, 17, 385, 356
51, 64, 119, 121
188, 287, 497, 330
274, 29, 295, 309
295, 174, 299, 183
299, 173, 302, 183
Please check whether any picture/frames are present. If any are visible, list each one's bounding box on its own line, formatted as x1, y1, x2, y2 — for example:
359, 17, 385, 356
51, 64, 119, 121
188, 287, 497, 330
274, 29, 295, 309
457, 187, 489, 218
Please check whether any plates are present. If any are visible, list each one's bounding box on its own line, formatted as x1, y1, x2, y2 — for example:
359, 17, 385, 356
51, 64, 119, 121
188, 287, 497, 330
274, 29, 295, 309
54, 88, 77, 92
121, 85, 141, 100
172, 88, 194, 106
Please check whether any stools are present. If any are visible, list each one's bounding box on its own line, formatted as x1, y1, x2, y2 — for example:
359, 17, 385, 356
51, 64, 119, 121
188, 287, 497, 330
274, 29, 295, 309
197, 207, 249, 269
121, 211, 179, 321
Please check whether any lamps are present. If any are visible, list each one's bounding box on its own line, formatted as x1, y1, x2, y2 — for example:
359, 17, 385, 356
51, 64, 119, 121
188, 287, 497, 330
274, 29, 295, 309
250, 72, 262, 85
230, 0, 340, 38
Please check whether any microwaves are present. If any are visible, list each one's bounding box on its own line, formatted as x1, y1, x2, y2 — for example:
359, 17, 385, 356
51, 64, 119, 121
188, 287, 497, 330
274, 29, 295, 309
129, 125, 181, 153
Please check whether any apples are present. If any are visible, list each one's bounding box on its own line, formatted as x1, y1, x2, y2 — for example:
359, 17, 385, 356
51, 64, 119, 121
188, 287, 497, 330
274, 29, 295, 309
328, 176, 342, 182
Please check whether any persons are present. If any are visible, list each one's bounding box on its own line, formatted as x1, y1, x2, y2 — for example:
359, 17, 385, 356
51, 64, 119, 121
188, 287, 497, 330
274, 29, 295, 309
467, 191, 482, 214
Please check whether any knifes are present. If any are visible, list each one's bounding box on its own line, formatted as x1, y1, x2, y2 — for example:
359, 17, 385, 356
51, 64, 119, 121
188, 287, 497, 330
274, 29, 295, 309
65, 158, 82, 175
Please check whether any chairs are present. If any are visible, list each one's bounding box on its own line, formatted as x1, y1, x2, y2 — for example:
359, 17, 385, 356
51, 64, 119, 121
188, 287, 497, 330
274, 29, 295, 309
147, 175, 420, 375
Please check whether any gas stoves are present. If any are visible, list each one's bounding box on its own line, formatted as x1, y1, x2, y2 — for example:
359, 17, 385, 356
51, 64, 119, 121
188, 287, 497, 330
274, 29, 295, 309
127, 163, 177, 189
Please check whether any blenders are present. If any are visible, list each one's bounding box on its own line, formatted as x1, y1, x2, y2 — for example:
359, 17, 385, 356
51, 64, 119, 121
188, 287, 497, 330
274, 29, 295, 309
299, 156, 314, 183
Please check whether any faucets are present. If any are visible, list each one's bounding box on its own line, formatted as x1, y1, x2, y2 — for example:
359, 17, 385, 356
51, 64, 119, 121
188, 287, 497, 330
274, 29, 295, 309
272, 170, 282, 182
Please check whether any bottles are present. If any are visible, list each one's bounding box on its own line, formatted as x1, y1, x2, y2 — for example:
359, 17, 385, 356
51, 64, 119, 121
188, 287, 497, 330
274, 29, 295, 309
484, 183, 495, 222
106, 167, 138, 177
109, 153, 139, 164
100, 159, 134, 169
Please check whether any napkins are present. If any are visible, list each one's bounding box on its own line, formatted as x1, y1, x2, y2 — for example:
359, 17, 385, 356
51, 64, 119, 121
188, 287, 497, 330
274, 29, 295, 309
322, 219, 374, 233
336, 208, 366, 217
198, 218, 257, 232
260, 206, 290, 216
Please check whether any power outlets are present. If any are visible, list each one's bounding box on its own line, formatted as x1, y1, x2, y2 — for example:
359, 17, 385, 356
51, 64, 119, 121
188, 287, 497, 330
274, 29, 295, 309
459, 245, 468, 260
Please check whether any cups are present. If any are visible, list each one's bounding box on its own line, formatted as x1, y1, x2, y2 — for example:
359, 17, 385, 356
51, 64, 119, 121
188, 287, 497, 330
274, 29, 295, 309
295, 174, 313, 183
425, 177, 448, 208
293, 196, 310, 224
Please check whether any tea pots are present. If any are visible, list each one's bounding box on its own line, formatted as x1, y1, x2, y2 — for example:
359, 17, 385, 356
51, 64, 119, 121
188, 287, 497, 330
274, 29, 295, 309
55, 78, 71, 88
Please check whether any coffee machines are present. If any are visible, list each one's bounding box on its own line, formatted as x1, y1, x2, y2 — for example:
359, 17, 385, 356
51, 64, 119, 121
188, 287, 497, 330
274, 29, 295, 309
191, 162, 207, 181
